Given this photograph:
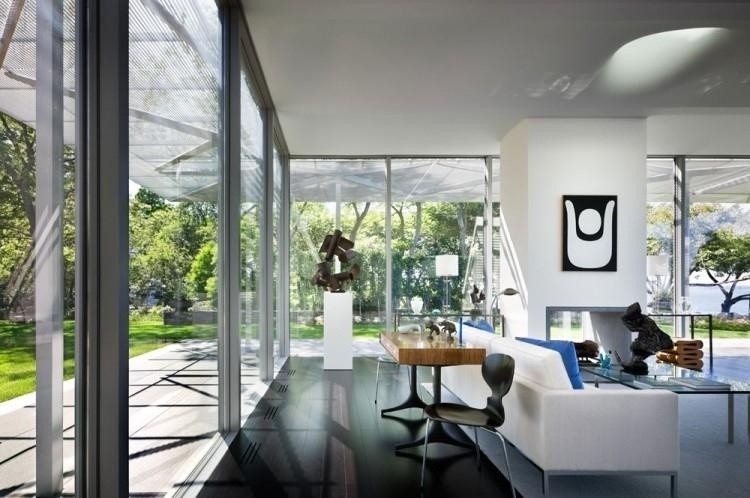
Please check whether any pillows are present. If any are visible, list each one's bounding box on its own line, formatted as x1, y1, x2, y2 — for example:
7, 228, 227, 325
475, 318, 584, 390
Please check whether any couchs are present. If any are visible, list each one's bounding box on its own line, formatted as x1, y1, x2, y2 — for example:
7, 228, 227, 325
432, 315, 678, 497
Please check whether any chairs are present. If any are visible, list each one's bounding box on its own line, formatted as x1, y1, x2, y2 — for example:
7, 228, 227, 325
420, 348, 518, 498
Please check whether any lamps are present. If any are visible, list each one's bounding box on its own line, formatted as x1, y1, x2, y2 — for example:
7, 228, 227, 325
491, 286, 520, 324
434, 254, 459, 321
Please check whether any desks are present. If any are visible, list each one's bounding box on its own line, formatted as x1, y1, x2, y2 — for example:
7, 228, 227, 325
380, 330, 487, 451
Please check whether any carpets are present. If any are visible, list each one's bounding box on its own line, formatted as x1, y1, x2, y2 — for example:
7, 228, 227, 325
421, 356, 750, 498
